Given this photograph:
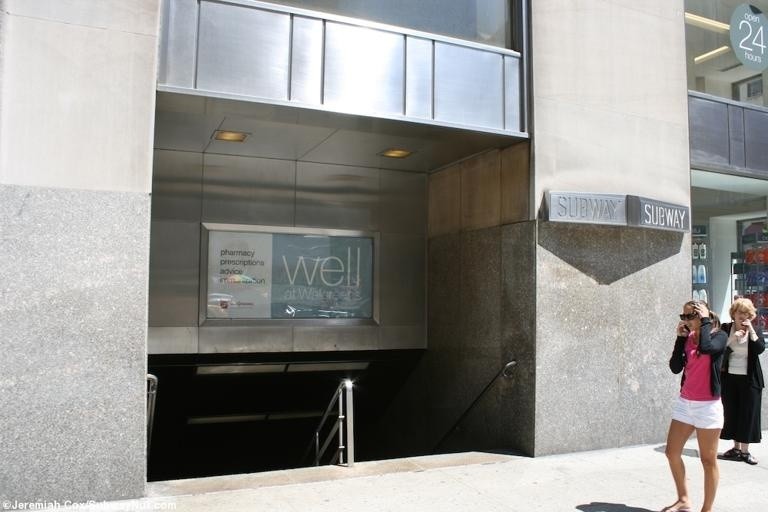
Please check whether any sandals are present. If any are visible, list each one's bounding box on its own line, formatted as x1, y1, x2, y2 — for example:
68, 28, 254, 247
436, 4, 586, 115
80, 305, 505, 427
724, 448, 741, 458
738, 452, 758, 465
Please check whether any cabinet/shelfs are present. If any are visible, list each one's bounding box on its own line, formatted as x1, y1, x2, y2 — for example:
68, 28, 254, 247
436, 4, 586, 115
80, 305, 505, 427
730, 231, 768, 335
692, 239, 713, 310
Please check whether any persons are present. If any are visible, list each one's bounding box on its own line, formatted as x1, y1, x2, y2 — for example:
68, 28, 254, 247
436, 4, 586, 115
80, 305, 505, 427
715, 298, 764, 465
660, 299, 730, 511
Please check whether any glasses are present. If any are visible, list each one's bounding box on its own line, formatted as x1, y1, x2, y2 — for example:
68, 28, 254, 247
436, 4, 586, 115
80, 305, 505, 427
680, 313, 698, 320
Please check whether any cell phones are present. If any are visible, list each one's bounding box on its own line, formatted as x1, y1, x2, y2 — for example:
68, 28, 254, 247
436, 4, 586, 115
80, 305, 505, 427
683, 324, 691, 333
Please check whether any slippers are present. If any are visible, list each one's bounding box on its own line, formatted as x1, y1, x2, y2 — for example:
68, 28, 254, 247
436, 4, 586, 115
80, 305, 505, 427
660, 505, 693, 512
